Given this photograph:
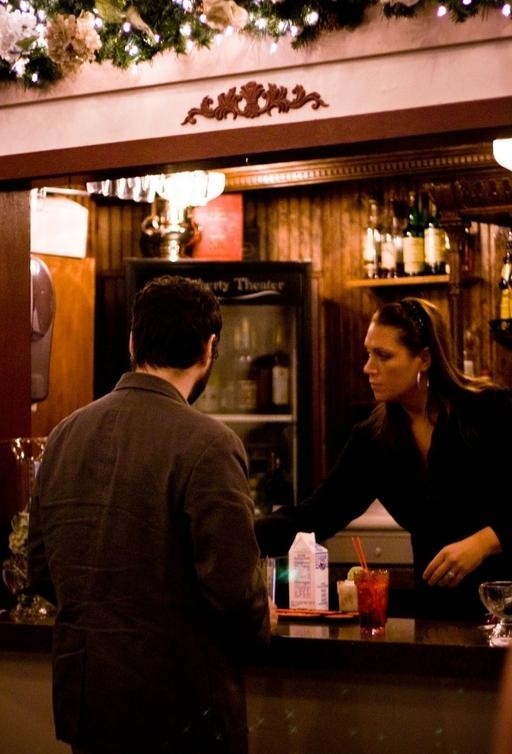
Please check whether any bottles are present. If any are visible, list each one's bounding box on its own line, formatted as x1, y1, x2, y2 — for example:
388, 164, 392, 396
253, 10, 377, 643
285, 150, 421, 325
498, 253, 512, 333
365, 190, 447, 278
222, 316, 289, 413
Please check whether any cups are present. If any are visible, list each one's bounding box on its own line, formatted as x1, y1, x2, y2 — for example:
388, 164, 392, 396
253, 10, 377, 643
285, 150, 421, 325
487, 620, 512, 647
337, 570, 388, 636
478, 580, 512, 620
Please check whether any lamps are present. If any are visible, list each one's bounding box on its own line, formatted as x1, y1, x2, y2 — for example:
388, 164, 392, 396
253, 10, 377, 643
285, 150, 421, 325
137, 170, 230, 260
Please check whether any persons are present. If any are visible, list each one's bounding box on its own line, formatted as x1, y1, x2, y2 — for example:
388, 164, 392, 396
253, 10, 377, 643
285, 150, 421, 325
28, 278, 274, 753
254, 298, 512, 625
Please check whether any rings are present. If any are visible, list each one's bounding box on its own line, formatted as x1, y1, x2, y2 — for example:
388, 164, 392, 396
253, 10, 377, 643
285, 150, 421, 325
447, 570, 456, 579
